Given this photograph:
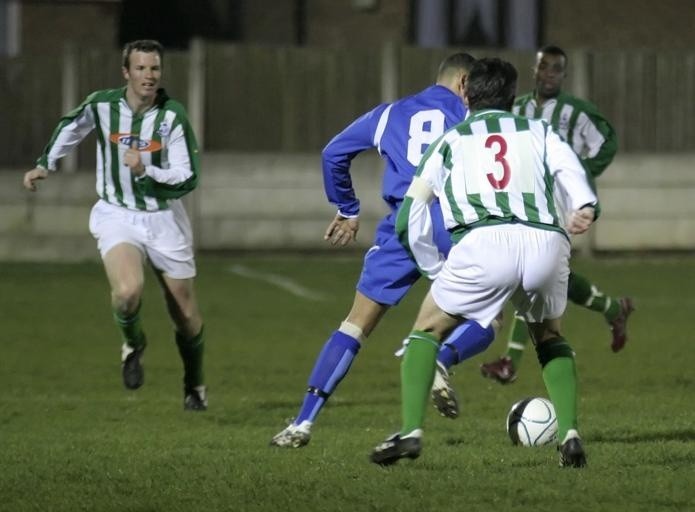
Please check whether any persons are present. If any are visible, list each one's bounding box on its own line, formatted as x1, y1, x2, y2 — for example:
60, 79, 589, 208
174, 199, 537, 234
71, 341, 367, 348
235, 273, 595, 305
270, 52, 503, 447
372, 56, 600, 468
480, 47, 636, 384
22, 39, 211, 410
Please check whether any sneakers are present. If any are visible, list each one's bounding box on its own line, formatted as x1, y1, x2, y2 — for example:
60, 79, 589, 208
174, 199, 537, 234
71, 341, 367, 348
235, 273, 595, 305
556, 429, 586, 468
183, 368, 208, 411
370, 429, 424, 466
480, 355, 519, 385
431, 358, 459, 420
121, 333, 147, 390
609, 297, 636, 352
270, 419, 313, 449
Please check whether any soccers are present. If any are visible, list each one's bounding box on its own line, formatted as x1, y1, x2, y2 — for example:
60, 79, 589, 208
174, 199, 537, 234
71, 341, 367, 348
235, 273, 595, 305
506, 397, 561, 448
